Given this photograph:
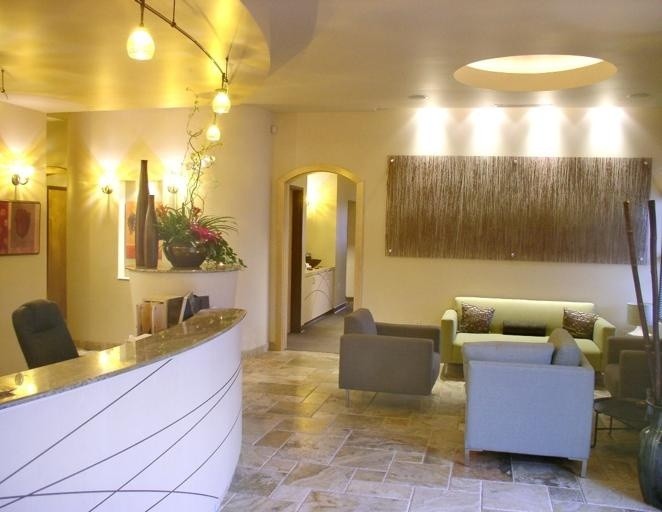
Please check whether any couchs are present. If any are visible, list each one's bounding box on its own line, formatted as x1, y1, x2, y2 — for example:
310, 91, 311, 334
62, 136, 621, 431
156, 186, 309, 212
440, 296, 615, 374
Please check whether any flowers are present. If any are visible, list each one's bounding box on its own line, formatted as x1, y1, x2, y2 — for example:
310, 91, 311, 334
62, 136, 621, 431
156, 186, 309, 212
153, 201, 249, 268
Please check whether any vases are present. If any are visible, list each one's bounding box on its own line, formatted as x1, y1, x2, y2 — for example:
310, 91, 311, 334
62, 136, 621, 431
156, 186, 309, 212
163, 241, 204, 267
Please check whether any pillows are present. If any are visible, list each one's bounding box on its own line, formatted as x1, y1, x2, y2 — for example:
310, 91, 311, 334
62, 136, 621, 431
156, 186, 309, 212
562, 309, 596, 338
548, 328, 583, 366
502, 320, 547, 335
460, 341, 558, 363
459, 302, 495, 333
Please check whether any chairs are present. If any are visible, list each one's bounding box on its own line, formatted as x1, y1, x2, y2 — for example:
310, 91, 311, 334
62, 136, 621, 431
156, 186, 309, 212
603, 335, 661, 399
464, 357, 595, 476
339, 310, 441, 406
591, 398, 647, 448
13, 298, 77, 368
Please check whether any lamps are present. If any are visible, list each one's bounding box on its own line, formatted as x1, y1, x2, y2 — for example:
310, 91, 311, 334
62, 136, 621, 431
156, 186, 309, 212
199, 70, 231, 168
0, 165, 40, 255
627, 302, 651, 336
103, 186, 112, 195
126, 0, 154, 60
169, 186, 177, 193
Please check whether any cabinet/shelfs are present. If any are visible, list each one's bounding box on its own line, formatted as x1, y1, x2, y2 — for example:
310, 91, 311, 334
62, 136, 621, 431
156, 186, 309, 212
303, 266, 334, 325
